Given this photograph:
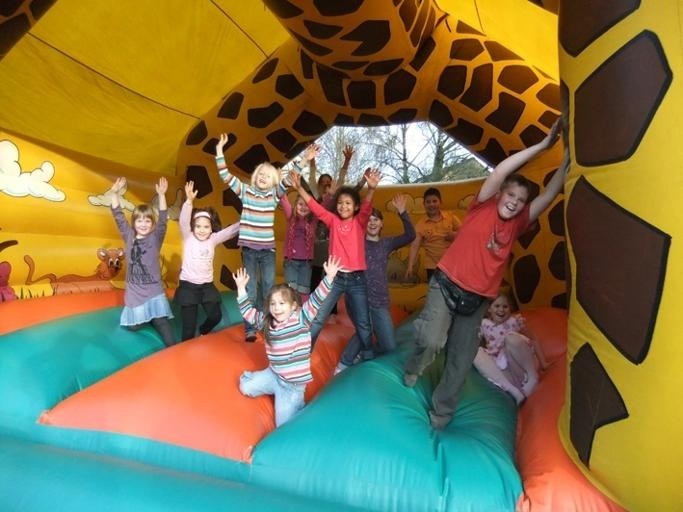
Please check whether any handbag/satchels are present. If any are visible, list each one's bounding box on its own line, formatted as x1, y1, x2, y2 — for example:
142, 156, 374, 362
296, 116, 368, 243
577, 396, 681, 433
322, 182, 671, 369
432, 267, 486, 317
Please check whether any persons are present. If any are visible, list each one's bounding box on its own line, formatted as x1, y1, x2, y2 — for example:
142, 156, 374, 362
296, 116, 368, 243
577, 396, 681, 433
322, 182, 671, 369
469, 289, 554, 404
400, 115, 572, 430
109, 174, 178, 346
403, 187, 461, 285
170, 180, 242, 345
213, 130, 416, 378
230, 255, 345, 427
